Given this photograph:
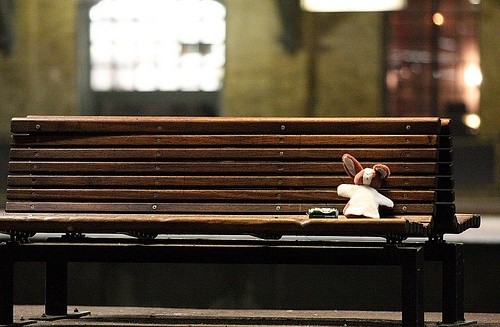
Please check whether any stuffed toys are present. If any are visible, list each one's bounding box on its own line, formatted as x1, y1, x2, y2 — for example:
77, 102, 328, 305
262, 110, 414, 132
337, 153, 394, 218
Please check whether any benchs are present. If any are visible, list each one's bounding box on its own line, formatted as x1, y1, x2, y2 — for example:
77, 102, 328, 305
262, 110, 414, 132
0, 115, 482, 327
